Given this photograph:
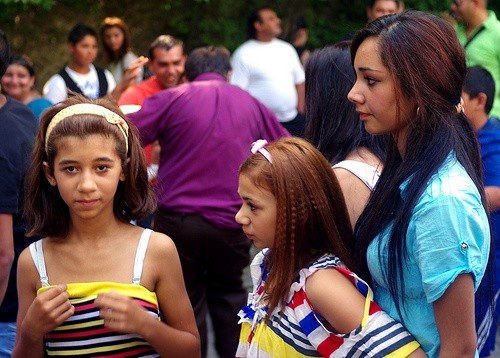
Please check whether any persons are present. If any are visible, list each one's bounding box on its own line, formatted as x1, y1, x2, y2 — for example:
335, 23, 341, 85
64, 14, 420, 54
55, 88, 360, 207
347, 10, 496, 358
0, 0, 500, 358
235, 137, 428, 358
11, 87, 200, 358
